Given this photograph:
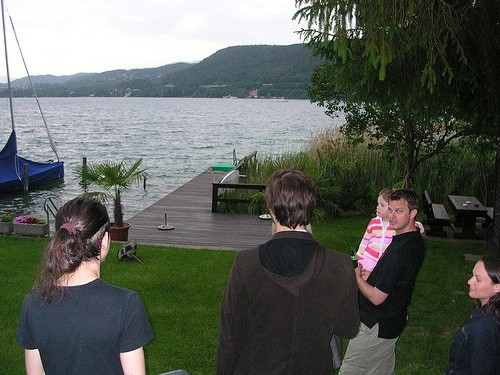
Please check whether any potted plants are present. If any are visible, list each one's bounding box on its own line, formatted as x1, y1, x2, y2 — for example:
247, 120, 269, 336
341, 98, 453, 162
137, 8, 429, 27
0, 211, 14, 235
72, 158, 150, 242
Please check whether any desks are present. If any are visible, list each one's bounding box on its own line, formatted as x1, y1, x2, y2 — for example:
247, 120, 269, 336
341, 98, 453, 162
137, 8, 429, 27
448, 195, 488, 240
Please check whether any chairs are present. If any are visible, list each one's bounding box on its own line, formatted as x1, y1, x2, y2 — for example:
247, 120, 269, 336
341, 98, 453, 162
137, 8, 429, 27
211, 148, 237, 182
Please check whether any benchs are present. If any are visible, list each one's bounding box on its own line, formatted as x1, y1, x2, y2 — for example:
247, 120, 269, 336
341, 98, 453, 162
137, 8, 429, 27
422, 190, 450, 237
481, 207, 494, 228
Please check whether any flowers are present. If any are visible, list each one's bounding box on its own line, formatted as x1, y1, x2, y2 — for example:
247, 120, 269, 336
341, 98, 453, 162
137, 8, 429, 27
13, 215, 46, 224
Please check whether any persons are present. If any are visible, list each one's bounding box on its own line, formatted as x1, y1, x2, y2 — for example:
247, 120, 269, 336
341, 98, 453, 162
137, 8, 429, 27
15, 198, 153, 375
338, 189, 425, 375
216, 169, 361, 375
446, 256, 500, 375
355, 188, 424, 281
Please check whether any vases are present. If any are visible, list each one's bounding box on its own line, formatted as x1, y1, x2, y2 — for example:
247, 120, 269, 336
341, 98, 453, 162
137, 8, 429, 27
13, 223, 48, 235
